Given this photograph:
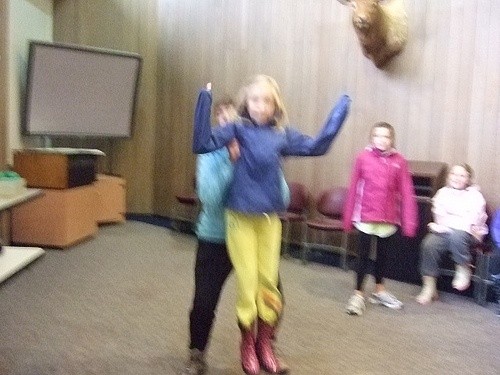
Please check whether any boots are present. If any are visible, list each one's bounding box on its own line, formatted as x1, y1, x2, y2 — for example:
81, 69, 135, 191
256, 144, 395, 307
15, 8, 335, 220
237, 320, 260, 375
257, 316, 280, 375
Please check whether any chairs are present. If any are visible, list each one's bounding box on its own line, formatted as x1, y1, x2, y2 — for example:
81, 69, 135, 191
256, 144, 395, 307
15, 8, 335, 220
439, 202, 500, 308
176, 174, 197, 232
278, 181, 359, 270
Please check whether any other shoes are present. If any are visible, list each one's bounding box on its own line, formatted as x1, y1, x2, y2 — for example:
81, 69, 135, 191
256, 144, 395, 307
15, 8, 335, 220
187, 348, 207, 375
346, 289, 365, 316
369, 289, 404, 311
452, 264, 471, 291
271, 339, 289, 374
414, 275, 438, 304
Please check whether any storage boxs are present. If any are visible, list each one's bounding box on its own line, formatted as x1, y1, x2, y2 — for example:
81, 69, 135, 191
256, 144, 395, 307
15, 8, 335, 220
11, 149, 128, 248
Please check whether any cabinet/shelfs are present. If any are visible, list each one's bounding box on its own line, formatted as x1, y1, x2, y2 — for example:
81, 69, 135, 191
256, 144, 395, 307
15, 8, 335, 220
0, 188, 45, 282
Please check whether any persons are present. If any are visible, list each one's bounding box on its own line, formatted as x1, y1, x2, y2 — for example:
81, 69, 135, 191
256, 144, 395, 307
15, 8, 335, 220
193, 76, 352, 375
187, 97, 289, 375
345, 122, 417, 316
413, 161, 489, 305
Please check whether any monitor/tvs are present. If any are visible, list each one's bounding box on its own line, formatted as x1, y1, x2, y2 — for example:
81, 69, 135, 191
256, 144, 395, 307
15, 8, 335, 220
21, 40, 143, 139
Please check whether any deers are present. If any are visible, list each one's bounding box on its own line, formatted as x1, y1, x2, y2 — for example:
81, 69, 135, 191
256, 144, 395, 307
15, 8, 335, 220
337, 0, 410, 69
